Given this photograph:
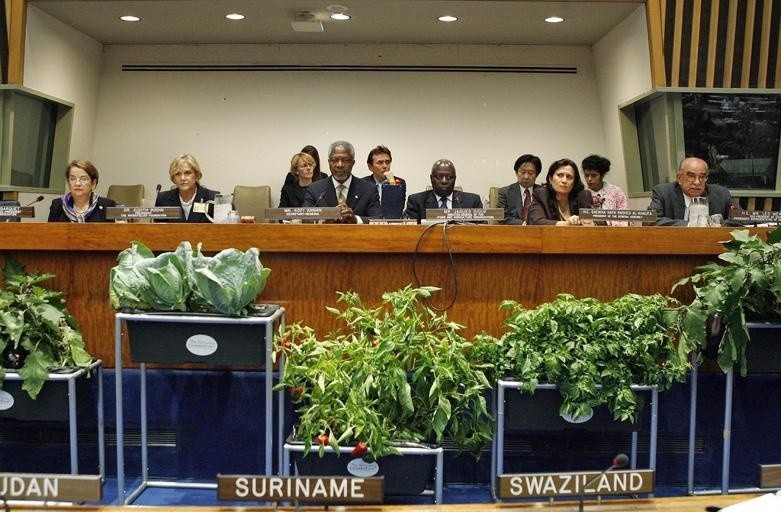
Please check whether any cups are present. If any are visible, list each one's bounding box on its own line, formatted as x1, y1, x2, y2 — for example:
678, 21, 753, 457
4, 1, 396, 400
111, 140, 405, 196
689, 196, 710, 226
228, 211, 240, 224
697, 214, 710, 228
204, 193, 233, 223
711, 213, 724, 228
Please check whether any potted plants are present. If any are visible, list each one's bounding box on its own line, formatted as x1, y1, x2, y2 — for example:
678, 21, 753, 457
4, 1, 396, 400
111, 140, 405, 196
672, 227, 781, 378
109, 241, 274, 370
471, 292, 695, 429
274, 284, 495, 491
0, 271, 95, 420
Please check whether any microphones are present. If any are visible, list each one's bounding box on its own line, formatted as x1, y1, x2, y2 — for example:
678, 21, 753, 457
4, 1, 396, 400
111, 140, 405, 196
25, 194, 44, 207
148, 183, 162, 222
576, 452, 630, 512
311, 191, 327, 208
453, 191, 464, 208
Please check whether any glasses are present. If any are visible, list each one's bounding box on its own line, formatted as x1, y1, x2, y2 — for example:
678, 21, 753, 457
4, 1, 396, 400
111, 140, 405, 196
67, 175, 93, 186
683, 173, 708, 183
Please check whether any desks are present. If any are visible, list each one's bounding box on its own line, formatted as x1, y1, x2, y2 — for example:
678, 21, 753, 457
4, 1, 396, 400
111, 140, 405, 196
2, 219, 773, 371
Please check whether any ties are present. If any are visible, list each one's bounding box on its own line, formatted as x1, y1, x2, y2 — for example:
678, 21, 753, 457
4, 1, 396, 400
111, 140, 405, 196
523, 189, 534, 220
439, 198, 447, 210
378, 182, 383, 204
337, 185, 346, 204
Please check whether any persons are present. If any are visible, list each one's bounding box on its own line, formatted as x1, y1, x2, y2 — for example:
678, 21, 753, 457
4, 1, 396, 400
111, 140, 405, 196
48, 159, 117, 222
641, 156, 745, 227
300, 141, 383, 224
154, 153, 220, 223
358, 145, 406, 212
497, 153, 543, 225
528, 158, 609, 227
581, 153, 634, 227
277, 144, 328, 225
403, 158, 488, 226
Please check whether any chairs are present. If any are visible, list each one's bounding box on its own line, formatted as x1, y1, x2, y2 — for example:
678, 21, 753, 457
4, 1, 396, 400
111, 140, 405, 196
106, 184, 145, 222
489, 186, 503, 225
426, 183, 465, 199
232, 185, 272, 222
